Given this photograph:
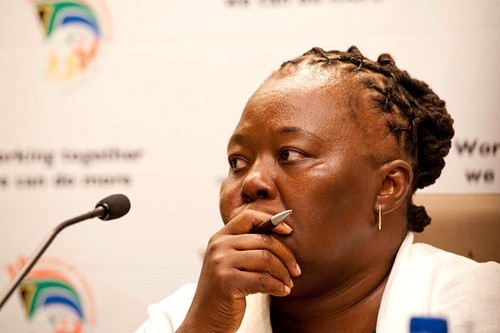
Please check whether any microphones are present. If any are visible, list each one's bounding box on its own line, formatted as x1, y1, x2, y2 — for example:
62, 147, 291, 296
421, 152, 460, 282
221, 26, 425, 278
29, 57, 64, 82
0, 193, 131, 309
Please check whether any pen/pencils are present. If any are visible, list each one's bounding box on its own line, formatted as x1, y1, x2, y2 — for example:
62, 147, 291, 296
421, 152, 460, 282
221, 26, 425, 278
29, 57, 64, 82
258, 209, 294, 233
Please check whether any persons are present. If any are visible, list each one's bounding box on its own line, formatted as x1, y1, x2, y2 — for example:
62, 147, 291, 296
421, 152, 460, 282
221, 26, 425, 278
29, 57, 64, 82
134, 44, 500, 333
